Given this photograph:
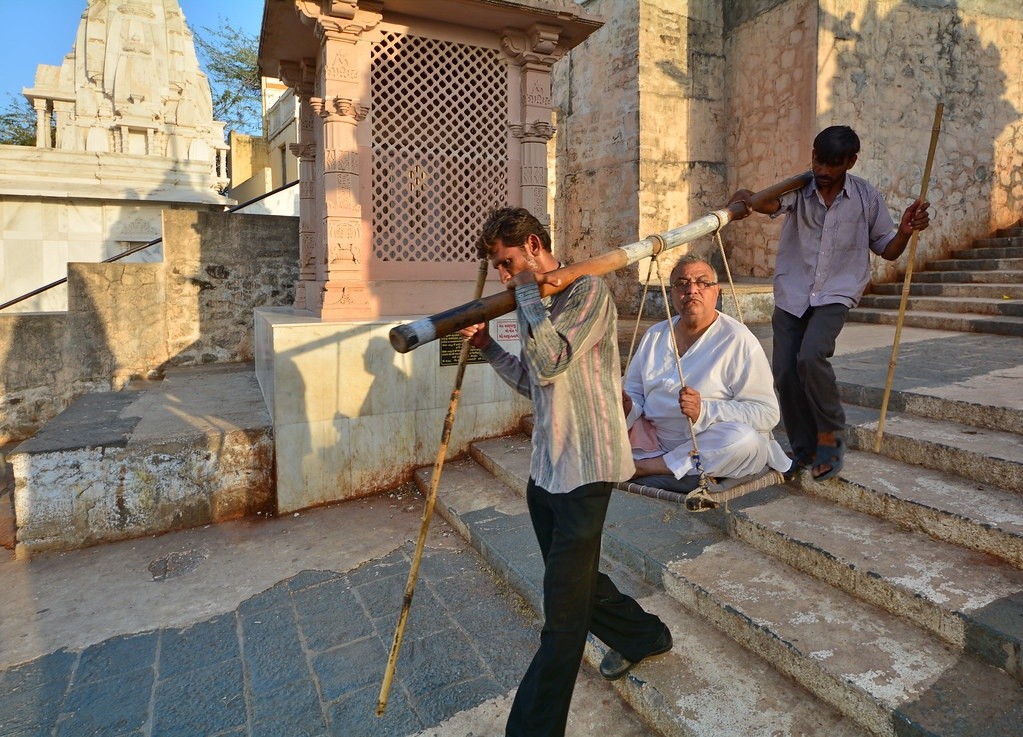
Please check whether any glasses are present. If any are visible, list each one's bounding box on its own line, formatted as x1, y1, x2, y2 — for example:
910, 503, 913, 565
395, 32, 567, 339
672, 280, 715, 292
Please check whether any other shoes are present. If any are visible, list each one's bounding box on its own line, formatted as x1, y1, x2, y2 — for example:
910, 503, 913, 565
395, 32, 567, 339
598, 622, 673, 680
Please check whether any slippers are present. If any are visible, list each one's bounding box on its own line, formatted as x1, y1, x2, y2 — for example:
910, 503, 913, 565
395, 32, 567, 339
782, 452, 815, 476
812, 437, 846, 482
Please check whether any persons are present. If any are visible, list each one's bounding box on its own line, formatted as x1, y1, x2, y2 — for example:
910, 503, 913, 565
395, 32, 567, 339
459, 207, 673, 737
725, 126, 930, 482
621, 254, 792, 479
